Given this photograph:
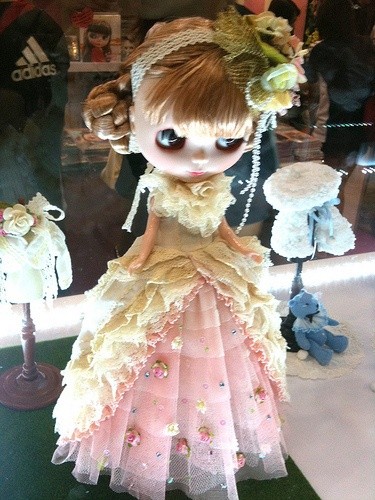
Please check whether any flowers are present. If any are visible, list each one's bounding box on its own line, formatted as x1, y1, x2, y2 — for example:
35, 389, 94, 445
240, 11, 308, 93
0, 204, 39, 237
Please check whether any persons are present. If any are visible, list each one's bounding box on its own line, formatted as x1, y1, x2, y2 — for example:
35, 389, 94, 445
51, 10, 316, 500
230, 0, 375, 216
0, 0, 70, 242
81, 19, 113, 63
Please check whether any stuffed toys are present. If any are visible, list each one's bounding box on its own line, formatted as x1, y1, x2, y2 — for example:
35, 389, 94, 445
288, 290, 348, 366
